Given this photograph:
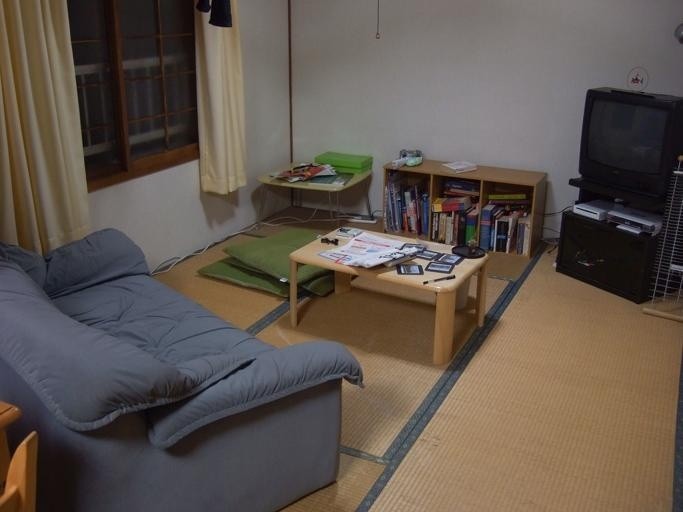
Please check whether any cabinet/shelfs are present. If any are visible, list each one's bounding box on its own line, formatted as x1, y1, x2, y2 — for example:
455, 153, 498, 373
382, 158, 548, 260
556, 178, 683, 304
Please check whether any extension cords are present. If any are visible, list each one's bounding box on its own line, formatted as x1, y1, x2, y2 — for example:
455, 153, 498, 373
347, 218, 378, 223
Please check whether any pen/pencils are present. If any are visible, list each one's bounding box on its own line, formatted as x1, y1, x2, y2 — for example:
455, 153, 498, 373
423, 274, 455, 285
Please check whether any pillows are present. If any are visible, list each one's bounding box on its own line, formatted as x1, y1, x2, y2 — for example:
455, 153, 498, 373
198, 226, 360, 298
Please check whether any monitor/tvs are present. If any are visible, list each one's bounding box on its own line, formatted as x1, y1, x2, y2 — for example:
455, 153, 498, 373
579, 87, 683, 198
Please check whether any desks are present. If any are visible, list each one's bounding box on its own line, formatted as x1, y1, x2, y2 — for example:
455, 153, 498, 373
0, 400, 22, 496
256, 163, 374, 231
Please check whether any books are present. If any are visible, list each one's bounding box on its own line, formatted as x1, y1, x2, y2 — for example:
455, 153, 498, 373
308, 172, 354, 187
384, 172, 532, 256
442, 157, 478, 174
383, 253, 417, 268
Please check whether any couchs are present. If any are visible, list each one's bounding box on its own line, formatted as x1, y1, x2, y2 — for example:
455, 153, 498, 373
0, 227, 365, 512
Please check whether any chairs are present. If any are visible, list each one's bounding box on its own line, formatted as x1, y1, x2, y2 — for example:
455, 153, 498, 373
0, 431, 39, 512
